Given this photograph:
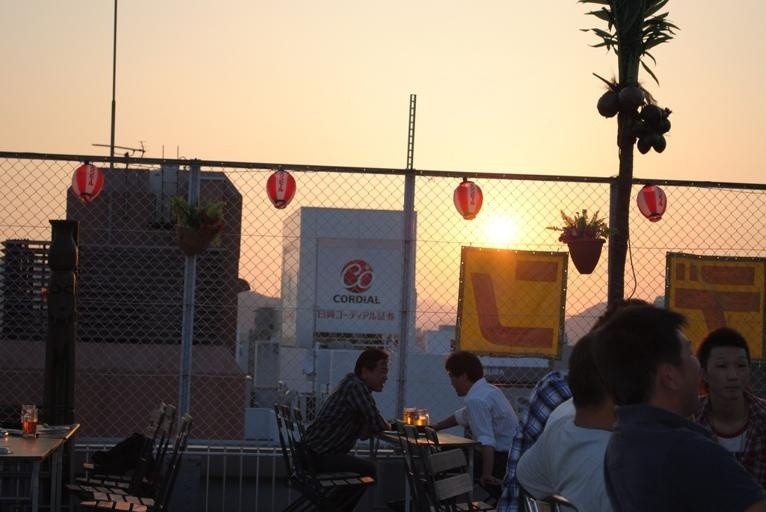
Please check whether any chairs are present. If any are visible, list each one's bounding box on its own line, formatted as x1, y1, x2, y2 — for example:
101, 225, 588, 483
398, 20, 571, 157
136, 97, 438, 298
67, 398, 195, 511
272, 400, 579, 512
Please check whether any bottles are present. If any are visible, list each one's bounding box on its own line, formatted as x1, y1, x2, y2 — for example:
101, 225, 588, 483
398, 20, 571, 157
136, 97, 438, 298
414, 409, 430, 426
0, 431, 9, 448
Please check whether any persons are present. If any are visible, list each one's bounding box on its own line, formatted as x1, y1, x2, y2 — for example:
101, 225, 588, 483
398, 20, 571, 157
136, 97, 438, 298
497, 299, 766, 511
415, 352, 518, 500
295, 348, 392, 512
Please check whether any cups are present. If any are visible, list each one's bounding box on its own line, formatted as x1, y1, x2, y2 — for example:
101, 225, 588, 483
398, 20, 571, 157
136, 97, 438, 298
403, 406, 416, 424
20, 403, 38, 435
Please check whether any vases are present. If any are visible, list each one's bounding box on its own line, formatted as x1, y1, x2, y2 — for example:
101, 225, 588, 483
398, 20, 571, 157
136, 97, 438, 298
177, 223, 210, 258
564, 238, 609, 276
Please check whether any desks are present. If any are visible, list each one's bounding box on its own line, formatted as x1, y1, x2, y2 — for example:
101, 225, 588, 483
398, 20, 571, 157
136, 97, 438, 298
1, 418, 80, 511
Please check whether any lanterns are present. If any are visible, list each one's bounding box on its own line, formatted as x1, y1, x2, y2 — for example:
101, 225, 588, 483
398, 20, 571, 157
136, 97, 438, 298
453, 181, 483, 221
266, 170, 297, 209
638, 184, 667, 222
71, 163, 105, 202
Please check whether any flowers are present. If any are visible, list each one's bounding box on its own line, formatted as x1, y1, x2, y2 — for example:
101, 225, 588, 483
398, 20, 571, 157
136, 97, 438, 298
547, 207, 625, 242
165, 193, 228, 250
